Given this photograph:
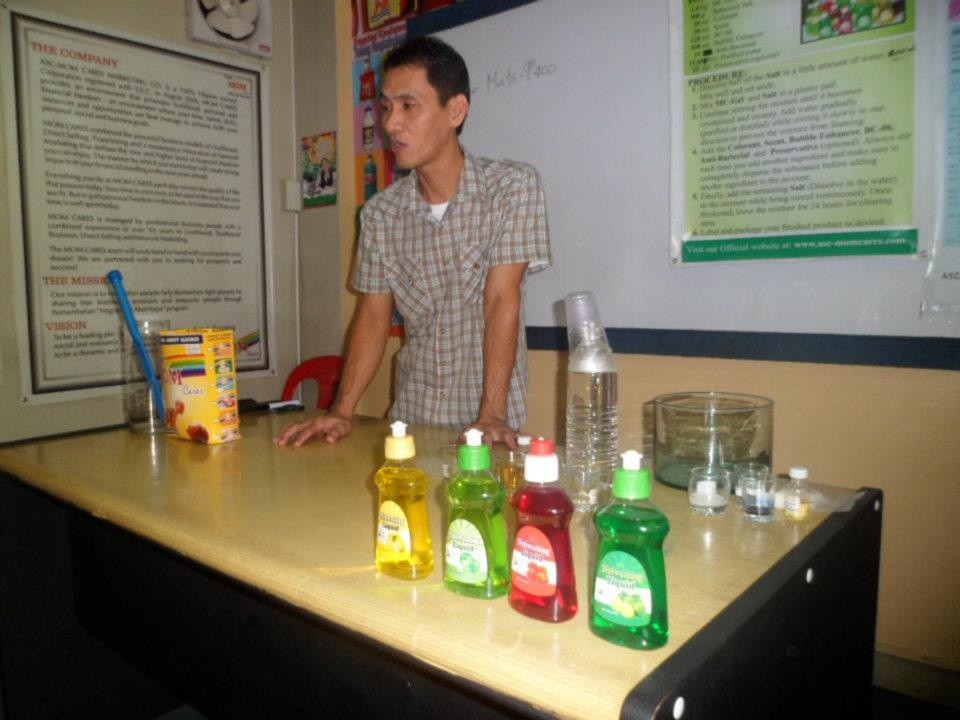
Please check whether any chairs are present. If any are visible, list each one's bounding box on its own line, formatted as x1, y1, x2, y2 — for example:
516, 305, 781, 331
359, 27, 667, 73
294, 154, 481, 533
282, 355, 344, 410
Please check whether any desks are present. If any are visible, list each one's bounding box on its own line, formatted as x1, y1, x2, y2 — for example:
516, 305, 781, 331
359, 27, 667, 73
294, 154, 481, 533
1, 408, 884, 720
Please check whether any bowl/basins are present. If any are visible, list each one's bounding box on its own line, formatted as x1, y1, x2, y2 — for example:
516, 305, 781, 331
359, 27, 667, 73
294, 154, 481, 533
654, 392, 774, 493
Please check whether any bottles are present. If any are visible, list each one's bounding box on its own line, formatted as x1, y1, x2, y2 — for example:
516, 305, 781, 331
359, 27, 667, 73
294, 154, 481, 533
445, 429, 508, 599
784, 463, 812, 522
567, 322, 618, 474
374, 421, 435, 579
509, 438, 580, 624
589, 450, 671, 650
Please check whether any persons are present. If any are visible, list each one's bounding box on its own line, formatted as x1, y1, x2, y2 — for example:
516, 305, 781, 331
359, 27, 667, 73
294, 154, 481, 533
271, 38, 554, 450
314, 158, 334, 195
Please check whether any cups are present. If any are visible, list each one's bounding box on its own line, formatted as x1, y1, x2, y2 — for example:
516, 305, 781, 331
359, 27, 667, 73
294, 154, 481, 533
565, 293, 613, 364
741, 472, 777, 523
686, 467, 731, 517
494, 449, 525, 503
118, 320, 172, 434
